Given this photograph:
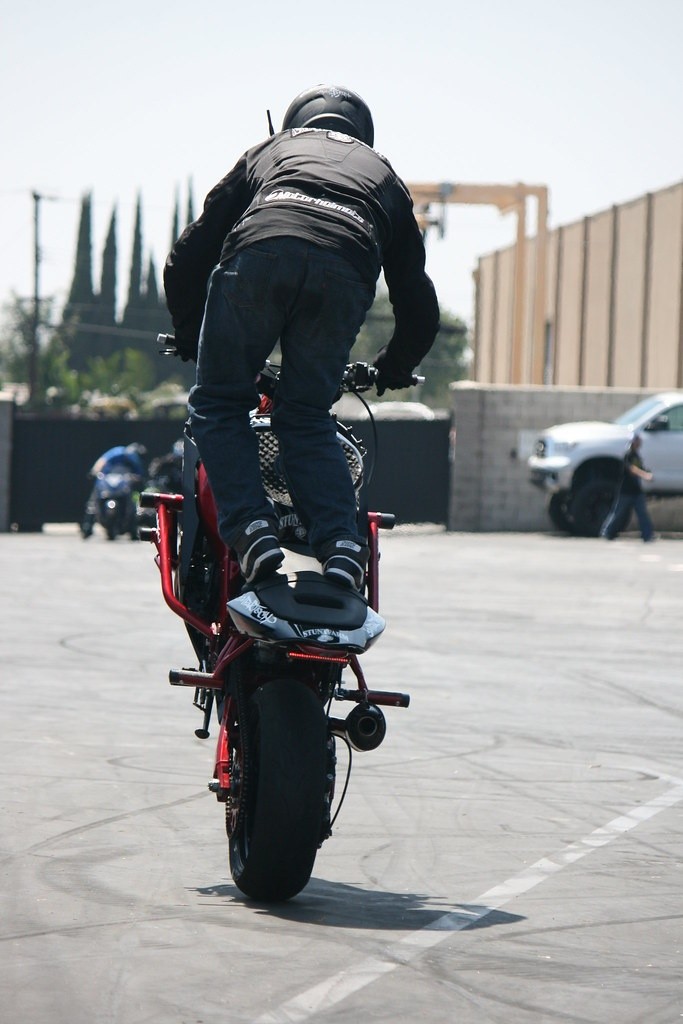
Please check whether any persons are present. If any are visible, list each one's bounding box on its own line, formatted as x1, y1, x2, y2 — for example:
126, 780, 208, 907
149, 439, 184, 495
601, 433, 661, 544
163, 83, 441, 602
82, 443, 147, 542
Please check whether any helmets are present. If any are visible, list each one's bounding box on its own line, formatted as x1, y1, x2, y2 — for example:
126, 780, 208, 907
281, 85, 375, 148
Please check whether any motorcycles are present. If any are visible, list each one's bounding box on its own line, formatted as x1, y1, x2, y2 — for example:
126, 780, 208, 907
138, 324, 427, 906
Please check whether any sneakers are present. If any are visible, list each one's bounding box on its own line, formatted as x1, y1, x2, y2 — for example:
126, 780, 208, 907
231, 520, 285, 583
320, 540, 371, 594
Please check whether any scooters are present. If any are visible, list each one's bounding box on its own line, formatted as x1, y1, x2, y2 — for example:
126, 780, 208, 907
98, 472, 134, 539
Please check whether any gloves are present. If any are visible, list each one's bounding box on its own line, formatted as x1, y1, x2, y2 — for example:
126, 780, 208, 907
371, 346, 415, 397
174, 318, 202, 362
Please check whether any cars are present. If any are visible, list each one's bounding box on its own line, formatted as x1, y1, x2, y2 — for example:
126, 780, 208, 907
527, 393, 683, 540
